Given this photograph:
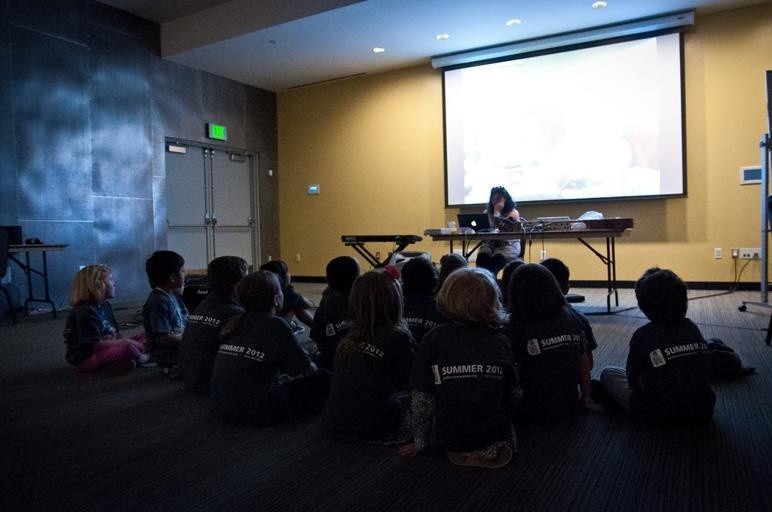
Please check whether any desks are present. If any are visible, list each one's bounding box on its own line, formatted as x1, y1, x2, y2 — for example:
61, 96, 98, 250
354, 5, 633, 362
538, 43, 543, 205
432, 230, 632, 315
0, 243, 70, 320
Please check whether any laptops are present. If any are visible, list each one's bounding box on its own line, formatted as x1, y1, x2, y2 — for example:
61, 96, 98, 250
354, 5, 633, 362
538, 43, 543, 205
455, 212, 500, 232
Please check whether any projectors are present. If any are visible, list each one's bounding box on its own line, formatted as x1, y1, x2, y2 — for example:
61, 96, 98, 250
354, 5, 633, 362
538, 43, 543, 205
539, 215, 569, 231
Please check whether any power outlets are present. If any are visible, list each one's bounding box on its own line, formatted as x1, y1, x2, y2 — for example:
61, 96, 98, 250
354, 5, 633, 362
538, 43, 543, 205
739, 248, 761, 260
731, 249, 739, 259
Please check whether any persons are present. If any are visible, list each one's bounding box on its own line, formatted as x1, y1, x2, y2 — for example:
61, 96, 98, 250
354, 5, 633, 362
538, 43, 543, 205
476, 187, 521, 275
64, 264, 153, 374
588, 267, 716, 436
308, 252, 597, 468
141, 251, 331, 428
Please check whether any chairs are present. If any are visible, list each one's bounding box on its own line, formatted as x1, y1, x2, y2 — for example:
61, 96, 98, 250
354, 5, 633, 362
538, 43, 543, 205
517, 216, 526, 263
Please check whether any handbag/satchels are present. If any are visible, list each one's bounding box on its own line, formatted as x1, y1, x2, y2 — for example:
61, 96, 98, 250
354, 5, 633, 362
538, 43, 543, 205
700, 338, 741, 383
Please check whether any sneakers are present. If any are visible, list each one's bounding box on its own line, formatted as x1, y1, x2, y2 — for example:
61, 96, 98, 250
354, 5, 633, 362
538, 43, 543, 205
449, 444, 513, 469
120, 359, 137, 375
